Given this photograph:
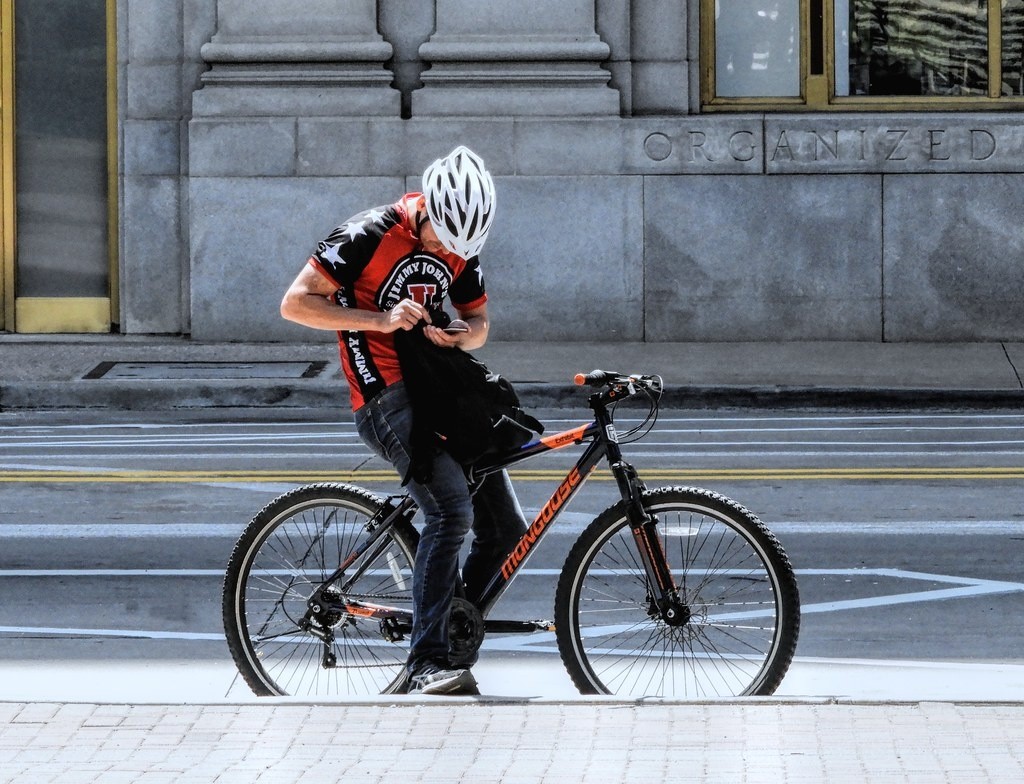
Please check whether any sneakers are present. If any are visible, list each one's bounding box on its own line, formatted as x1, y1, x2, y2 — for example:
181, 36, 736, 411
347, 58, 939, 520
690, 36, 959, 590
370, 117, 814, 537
407, 659, 476, 695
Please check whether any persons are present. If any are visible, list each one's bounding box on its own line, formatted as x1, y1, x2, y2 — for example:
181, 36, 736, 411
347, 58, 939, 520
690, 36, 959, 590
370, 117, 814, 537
282, 142, 529, 695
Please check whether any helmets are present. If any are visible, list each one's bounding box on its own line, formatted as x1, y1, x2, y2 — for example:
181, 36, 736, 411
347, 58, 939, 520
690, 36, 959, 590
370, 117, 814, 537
415, 145, 497, 261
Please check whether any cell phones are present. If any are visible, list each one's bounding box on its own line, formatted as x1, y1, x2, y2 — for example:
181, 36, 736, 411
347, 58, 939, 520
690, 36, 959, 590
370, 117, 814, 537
443, 328, 468, 333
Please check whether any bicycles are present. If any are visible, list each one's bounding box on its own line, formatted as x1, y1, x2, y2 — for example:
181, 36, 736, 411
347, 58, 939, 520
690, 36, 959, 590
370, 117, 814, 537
220, 367, 803, 695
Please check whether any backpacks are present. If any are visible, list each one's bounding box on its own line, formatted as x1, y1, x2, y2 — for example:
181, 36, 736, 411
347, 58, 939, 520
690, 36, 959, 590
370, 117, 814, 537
394, 311, 545, 486
